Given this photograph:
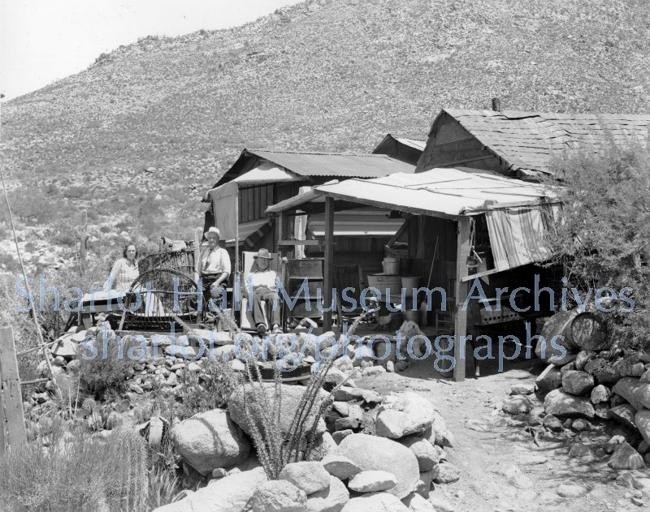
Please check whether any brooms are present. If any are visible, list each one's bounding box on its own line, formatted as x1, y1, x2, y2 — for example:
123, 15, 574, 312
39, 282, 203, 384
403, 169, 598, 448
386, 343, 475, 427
420, 233, 440, 326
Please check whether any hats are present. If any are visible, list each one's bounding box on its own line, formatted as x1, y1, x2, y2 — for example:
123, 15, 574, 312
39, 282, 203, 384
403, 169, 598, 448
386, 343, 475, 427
204, 226, 221, 241
253, 248, 274, 260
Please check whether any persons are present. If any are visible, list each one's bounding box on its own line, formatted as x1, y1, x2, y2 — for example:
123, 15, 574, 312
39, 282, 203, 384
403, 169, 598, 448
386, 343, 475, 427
185, 225, 232, 317
99, 241, 164, 316
244, 248, 283, 333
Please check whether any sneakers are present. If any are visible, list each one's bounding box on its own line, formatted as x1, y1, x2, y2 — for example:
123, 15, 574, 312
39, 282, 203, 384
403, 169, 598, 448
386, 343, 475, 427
257, 322, 269, 336
271, 327, 283, 334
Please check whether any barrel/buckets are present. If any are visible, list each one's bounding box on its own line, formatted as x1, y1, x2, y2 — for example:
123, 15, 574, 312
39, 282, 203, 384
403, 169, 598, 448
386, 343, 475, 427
403, 309, 420, 325
368, 273, 403, 295
382, 257, 400, 275
288, 259, 324, 320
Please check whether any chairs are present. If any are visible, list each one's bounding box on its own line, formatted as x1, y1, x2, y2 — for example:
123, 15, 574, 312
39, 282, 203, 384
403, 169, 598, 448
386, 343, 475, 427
237, 250, 281, 334
